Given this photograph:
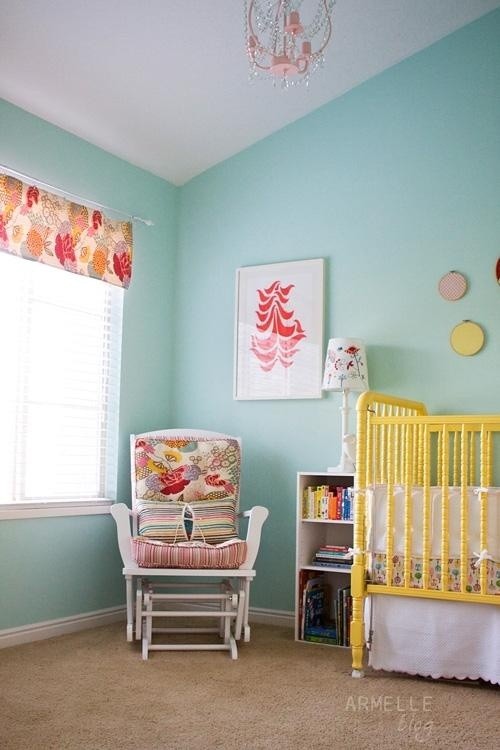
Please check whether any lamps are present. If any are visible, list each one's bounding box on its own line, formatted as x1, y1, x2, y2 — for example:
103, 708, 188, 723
322, 337, 370, 471
243, 0, 338, 91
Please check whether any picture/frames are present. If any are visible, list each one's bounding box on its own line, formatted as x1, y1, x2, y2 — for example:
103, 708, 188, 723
234, 257, 325, 401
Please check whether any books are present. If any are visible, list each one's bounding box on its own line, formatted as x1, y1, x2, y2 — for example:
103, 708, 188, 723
312, 544, 353, 569
298, 570, 351, 647
303, 483, 354, 521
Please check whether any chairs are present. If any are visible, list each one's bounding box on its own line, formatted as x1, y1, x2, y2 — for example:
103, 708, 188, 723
110, 427, 268, 660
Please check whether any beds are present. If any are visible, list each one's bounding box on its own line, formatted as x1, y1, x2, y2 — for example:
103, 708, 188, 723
351, 392, 500, 688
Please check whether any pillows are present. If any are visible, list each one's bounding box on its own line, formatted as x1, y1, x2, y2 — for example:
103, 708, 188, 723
135, 496, 239, 544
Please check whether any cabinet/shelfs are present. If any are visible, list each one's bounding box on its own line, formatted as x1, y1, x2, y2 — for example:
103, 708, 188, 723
294, 471, 356, 650
